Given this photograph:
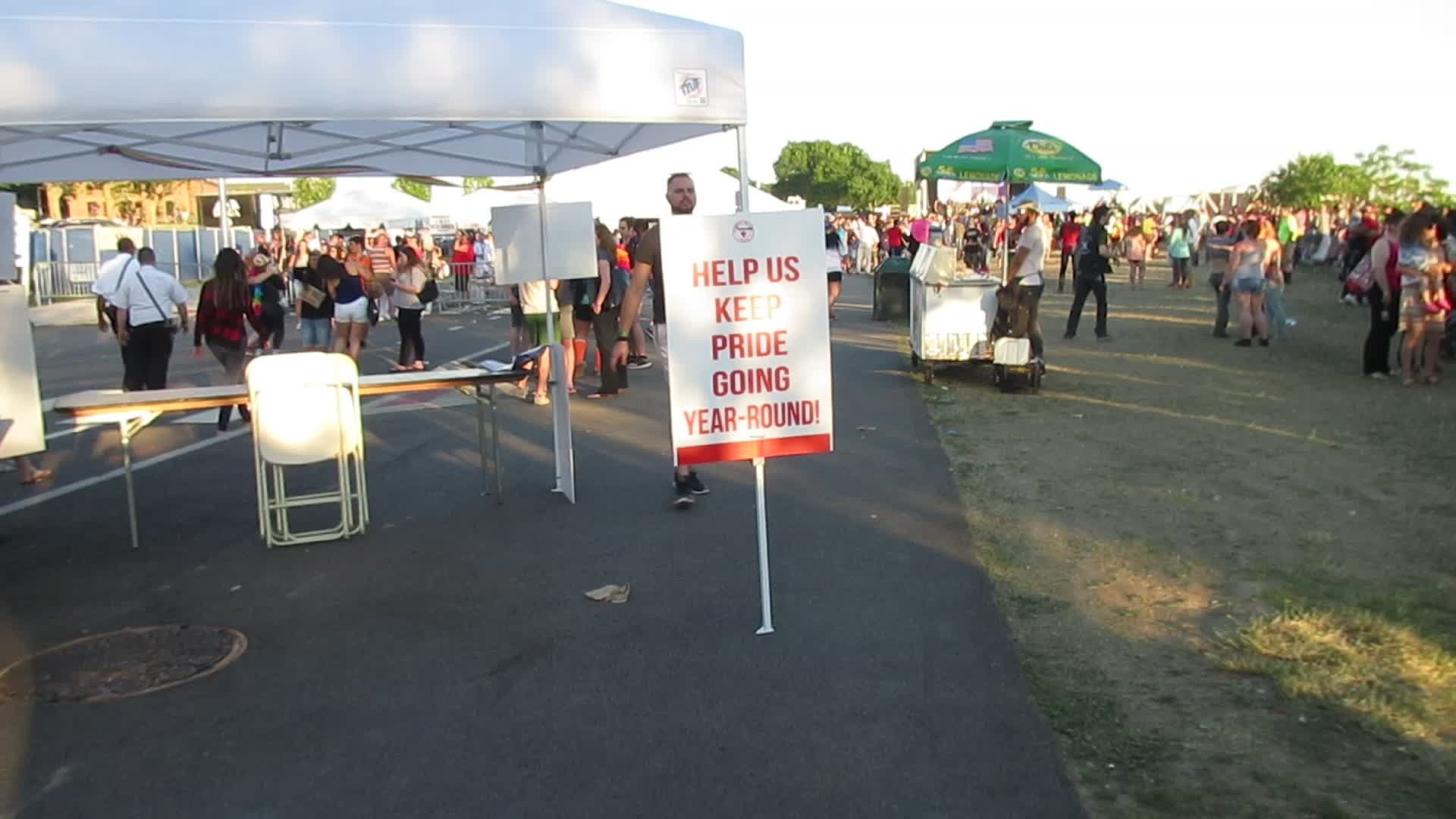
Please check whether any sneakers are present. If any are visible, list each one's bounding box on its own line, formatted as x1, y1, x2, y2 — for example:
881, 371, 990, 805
534, 392, 550, 405
673, 471, 710, 495
515, 387, 529, 399
627, 352, 652, 369
674, 473, 695, 504
586, 389, 614, 399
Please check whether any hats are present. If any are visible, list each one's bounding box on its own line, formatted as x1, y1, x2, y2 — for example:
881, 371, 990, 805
253, 253, 271, 267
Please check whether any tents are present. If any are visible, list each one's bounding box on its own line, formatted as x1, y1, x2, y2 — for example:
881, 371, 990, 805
1, 0, 788, 643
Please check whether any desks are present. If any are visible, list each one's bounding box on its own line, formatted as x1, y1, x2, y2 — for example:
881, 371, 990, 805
53, 364, 529, 553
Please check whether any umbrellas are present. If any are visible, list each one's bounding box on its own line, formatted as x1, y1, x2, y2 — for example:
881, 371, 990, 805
914, 119, 1105, 291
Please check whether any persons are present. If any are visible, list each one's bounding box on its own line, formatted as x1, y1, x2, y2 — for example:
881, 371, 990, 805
612, 170, 713, 507
192, 245, 272, 438
234, 196, 1456, 411
116, 249, 189, 387
92, 236, 140, 365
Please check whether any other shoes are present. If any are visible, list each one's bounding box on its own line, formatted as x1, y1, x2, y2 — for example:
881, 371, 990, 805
245, 345, 256, 357
413, 361, 425, 371
389, 364, 413, 371
1371, 364, 1441, 387
1063, 333, 1073, 339
1215, 328, 1270, 347
20, 469, 54, 486
256, 349, 267, 358
1097, 333, 1111, 341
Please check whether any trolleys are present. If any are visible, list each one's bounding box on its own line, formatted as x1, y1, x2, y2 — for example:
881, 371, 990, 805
908, 245, 1007, 386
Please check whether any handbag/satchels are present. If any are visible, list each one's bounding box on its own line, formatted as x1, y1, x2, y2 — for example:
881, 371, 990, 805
419, 280, 438, 303
367, 297, 380, 326
165, 318, 179, 334
